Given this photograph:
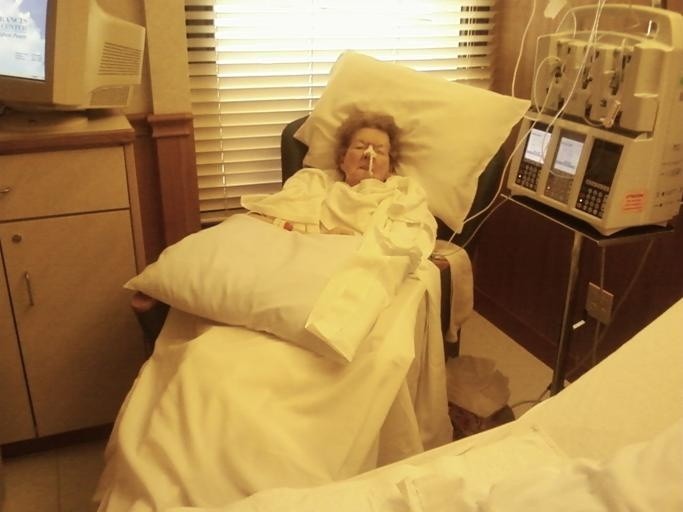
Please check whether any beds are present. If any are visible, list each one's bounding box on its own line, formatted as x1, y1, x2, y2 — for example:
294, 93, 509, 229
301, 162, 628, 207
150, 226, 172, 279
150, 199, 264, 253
229, 295, 680, 512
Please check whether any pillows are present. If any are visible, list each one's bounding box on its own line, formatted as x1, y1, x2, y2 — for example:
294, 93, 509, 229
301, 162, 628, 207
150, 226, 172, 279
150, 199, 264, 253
124, 214, 410, 364
302, 51, 532, 235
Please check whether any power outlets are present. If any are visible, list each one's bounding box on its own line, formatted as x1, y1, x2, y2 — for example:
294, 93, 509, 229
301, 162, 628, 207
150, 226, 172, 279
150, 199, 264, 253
586, 283, 614, 326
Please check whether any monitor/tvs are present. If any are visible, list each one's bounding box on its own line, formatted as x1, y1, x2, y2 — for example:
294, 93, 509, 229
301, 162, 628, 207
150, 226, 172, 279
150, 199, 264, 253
0, 0, 146, 133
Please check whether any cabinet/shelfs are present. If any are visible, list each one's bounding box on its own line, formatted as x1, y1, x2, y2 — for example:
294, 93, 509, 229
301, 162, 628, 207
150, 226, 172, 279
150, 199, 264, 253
0, 113, 156, 445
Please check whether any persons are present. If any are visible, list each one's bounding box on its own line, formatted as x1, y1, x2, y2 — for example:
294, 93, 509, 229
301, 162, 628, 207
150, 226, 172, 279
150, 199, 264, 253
333, 103, 404, 187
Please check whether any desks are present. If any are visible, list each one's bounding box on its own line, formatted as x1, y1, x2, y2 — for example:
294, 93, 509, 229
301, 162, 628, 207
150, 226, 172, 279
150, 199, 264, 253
472, 186, 675, 398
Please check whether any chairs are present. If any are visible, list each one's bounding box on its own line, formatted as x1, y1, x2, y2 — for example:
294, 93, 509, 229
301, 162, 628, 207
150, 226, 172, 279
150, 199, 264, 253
86, 117, 504, 507
